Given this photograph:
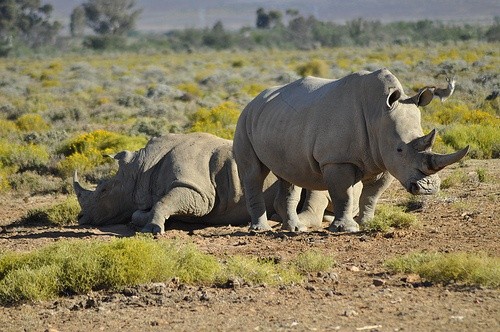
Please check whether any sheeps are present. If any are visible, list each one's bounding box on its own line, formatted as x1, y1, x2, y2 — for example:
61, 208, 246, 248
434, 73, 458, 102
